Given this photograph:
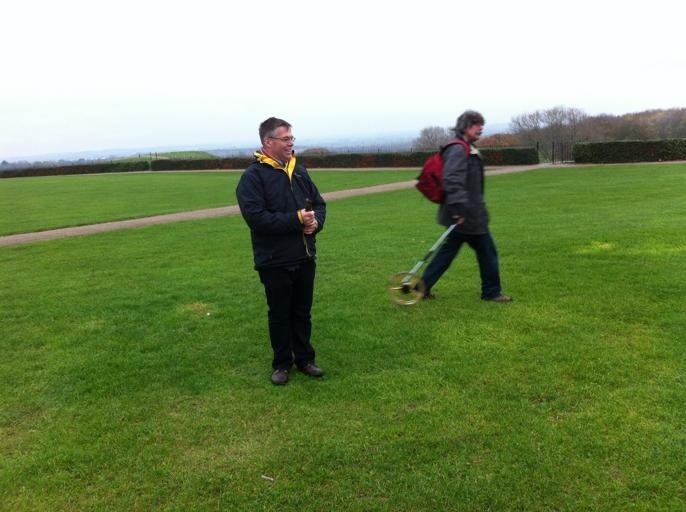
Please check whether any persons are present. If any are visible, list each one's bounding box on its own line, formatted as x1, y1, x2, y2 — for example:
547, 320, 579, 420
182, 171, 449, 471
235, 115, 327, 387
413, 108, 512, 304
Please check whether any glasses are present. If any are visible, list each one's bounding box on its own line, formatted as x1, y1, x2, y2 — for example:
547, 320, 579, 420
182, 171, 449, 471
269, 136, 295, 141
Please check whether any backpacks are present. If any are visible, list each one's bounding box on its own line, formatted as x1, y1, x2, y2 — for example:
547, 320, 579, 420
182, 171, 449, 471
416, 139, 470, 202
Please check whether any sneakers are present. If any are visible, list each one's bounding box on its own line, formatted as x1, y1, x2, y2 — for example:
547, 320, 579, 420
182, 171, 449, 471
271, 369, 288, 385
481, 295, 511, 301
301, 364, 320, 376
413, 288, 434, 299
